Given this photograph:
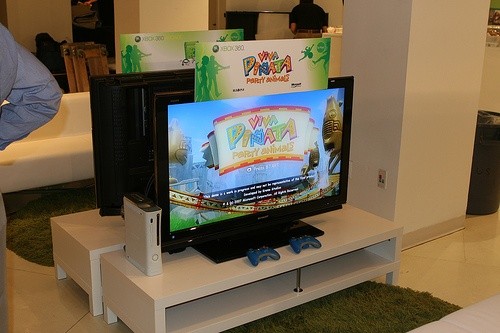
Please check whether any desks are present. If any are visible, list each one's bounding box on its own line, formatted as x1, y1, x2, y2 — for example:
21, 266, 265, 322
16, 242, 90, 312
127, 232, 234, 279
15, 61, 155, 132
322, 32, 343, 79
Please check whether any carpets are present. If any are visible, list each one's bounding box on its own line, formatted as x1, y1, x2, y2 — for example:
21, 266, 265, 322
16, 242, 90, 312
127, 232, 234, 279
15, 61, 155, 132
220, 280, 463, 333
5, 188, 98, 267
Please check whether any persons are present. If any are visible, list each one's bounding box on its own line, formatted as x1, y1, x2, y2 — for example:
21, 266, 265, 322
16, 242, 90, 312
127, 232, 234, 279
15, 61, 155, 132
289, 0, 328, 39
0, 21, 65, 332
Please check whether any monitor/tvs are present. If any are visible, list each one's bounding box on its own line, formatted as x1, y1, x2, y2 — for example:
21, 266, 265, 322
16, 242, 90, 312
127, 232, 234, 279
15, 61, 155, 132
89, 68, 196, 217
151, 75, 354, 264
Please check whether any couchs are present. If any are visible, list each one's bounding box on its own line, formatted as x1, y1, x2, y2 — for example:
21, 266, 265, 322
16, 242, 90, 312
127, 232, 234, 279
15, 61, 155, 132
1, 92, 96, 196
404, 294, 500, 333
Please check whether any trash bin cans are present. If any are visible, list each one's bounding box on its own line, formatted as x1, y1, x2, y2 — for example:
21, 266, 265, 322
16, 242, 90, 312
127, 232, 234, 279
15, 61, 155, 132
466, 110, 500, 215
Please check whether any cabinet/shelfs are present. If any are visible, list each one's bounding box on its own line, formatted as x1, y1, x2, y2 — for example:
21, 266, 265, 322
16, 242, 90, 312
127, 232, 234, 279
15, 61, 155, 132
50, 208, 127, 317
223, 11, 329, 40
100, 203, 403, 332
